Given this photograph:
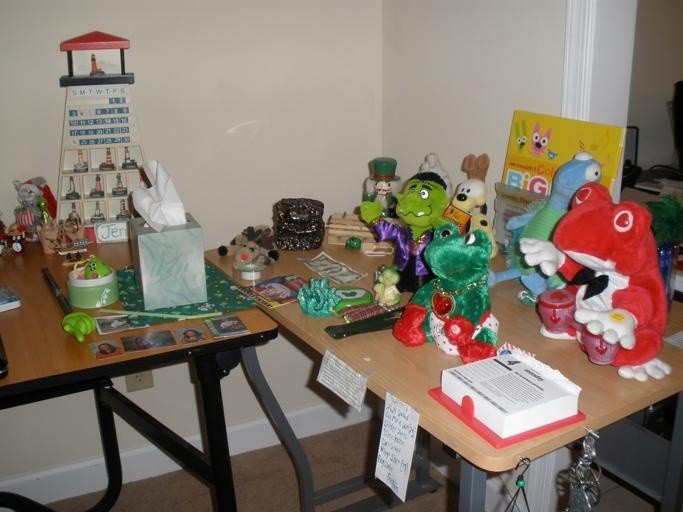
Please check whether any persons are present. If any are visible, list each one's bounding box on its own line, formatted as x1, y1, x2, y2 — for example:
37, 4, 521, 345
220, 318, 246, 332
183, 329, 204, 343
96, 344, 122, 360
134, 335, 148, 349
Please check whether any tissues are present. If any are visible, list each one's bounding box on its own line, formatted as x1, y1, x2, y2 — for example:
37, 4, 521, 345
128, 159, 209, 312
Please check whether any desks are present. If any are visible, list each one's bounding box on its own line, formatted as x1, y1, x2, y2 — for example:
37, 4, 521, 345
204, 227, 683, 512
0, 213, 278, 512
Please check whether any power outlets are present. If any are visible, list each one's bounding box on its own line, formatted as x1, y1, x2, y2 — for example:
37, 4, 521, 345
126, 370, 154, 393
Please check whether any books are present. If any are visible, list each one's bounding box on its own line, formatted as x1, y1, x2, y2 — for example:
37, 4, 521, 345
1, 285, 22, 314
429, 351, 586, 449
327, 213, 399, 239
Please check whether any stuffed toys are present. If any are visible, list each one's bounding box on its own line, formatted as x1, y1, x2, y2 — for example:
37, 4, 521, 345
358, 150, 682, 381
0, 176, 51, 258
219, 224, 281, 272
359, 149, 683, 382
